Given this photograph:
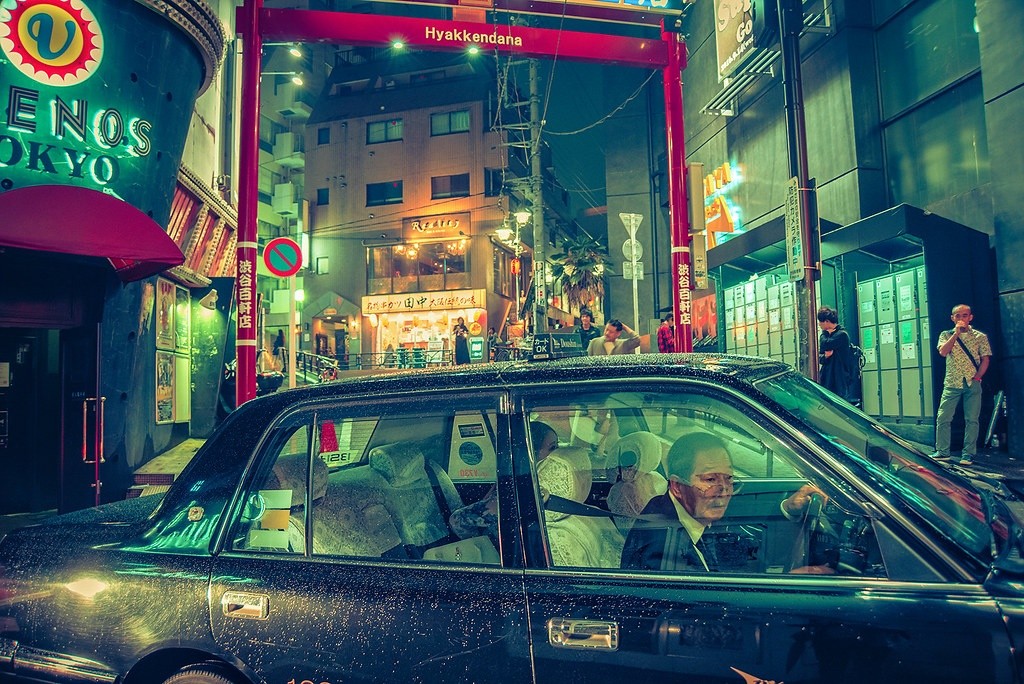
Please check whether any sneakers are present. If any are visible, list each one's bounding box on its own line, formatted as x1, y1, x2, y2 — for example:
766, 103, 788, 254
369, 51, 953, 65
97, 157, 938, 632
928, 452, 952, 461
959, 455, 976, 466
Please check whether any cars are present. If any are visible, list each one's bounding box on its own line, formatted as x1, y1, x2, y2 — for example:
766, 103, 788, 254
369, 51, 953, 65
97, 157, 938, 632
1, 350, 1023, 683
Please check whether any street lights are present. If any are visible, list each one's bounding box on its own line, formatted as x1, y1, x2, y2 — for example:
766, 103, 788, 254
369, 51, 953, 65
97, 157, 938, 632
494, 207, 532, 321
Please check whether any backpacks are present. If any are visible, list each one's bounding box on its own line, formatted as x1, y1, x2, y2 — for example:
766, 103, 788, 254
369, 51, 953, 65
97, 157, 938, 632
848, 342, 868, 383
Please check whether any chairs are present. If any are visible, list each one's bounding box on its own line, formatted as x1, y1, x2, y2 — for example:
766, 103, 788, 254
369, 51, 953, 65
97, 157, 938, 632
395, 347, 427, 369
266, 430, 669, 570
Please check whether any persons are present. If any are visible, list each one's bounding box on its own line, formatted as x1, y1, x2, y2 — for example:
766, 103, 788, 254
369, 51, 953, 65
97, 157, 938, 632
571, 309, 600, 350
816, 308, 851, 398
588, 317, 641, 356
488, 327, 499, 362
272, 329, 286, 372
620, 432, 836, 574
451, 317, 470, 365
385, 342, 409, 369
483, 421, 559, 500
929, 305, 992, 465
658, 313, 674, 353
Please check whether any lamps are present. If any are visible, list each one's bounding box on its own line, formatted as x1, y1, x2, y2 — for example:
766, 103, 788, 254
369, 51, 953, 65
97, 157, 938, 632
199, 288, 219, 311
260, 41, 305, 86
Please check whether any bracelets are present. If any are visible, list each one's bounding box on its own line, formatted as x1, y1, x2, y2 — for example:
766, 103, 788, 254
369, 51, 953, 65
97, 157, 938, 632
975, 373, 982, 383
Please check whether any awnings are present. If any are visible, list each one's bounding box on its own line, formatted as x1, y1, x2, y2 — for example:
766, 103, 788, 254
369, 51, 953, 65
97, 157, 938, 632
0, 185, 185, 285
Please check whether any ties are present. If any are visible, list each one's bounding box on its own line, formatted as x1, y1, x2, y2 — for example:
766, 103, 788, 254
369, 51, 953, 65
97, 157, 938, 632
702, 526, 720, 566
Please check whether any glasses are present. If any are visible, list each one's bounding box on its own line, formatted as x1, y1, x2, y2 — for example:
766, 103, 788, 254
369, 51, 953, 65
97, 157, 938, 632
680, 475, 742, 500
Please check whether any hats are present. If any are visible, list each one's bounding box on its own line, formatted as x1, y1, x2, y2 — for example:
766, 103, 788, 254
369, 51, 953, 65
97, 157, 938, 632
580, 309, 592, 316
